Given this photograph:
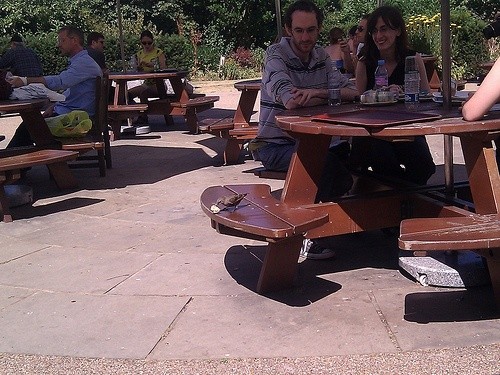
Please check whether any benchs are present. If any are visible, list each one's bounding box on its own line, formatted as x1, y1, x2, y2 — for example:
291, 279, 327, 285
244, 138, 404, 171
170, 96, 219, 114
201, 184, 330, 243
397, 214, 500, 256
56, 137, 105, 149
156, 94, 206, 101
108, 105, 148, 119
0, 146, 79, 171
198, 110, 257, 130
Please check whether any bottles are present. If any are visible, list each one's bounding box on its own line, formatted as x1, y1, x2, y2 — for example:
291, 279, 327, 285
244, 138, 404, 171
404, 56, 421, 111
327, 62, 341, 106
5, 68, 15, 88
131, 56, 137, 69
375, 60, 388, 91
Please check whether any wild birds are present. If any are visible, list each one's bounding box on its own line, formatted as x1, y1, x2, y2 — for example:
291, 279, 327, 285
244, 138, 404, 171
215, 191, 250, 214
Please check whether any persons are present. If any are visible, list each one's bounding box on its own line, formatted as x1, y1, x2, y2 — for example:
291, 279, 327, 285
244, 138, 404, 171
325, 15, 367, 80
127, 30, 167, 127
460, 55, 500, 122
352, 6, 436, 192
260, 5, 360, 262
5, 25, 104, 174
83, 32, 109, 72
0, 35, 41, 100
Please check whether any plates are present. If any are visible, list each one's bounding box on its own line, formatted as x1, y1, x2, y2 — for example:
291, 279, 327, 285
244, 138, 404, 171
360, 99, 398, 104
432, 90, 476, 102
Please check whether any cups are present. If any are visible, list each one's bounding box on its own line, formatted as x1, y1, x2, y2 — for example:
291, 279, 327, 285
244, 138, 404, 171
439, 80, 457, 97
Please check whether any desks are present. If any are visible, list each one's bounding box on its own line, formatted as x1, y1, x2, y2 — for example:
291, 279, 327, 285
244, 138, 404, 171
256, 99, 500, 295
0, 97, 76, 221
110, 70, 199, 141
224, 79, 262, 165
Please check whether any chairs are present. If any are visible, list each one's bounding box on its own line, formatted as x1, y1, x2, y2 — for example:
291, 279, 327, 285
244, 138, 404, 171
67, 73, 112, 178
